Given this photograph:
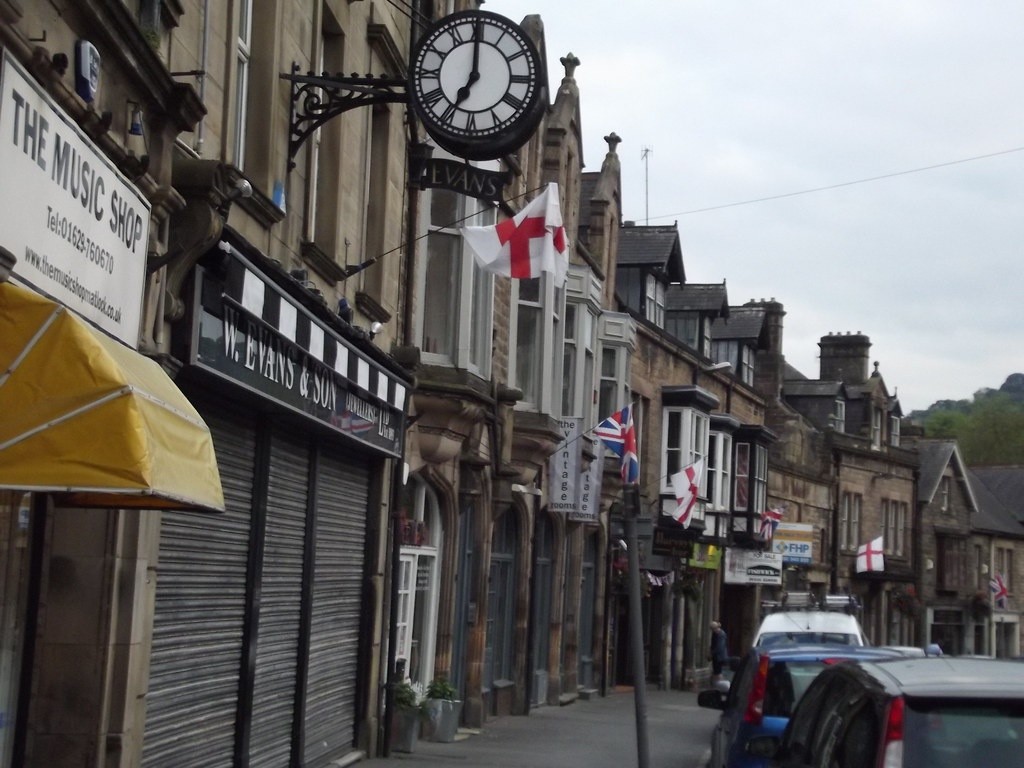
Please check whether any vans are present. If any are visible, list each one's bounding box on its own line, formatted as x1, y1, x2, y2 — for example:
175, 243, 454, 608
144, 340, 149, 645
752, 611, 871, 657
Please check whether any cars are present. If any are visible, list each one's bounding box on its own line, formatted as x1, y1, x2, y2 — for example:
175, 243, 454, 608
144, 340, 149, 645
694, 644, 909, 768
770, 655, 1024, 768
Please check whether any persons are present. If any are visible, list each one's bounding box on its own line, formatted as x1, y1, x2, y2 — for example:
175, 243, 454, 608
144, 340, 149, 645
707, 620, 727, 681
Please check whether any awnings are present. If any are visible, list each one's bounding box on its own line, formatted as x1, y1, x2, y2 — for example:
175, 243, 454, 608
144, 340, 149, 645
1, 281, 226, 516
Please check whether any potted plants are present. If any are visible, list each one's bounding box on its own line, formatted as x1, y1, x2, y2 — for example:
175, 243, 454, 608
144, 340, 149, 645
391, 683, 422, 753
423, 680, 462, 744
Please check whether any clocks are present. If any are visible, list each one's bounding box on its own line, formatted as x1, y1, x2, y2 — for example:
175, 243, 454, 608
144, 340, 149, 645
410, 8, 547, 161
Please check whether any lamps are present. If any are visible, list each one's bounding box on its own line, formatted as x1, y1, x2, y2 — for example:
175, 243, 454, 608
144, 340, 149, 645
370, 318, 383, 339
220, 176, 255, 210
696, 362, 733, 377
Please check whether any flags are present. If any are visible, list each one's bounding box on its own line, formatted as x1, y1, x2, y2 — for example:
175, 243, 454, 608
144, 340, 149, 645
987, 575, 1009, 611
760, 507, 784, 539
594, 403, 641, 486
461, 181, 569, 288
669, 460, 705, 529
855, 536, 884, 572
